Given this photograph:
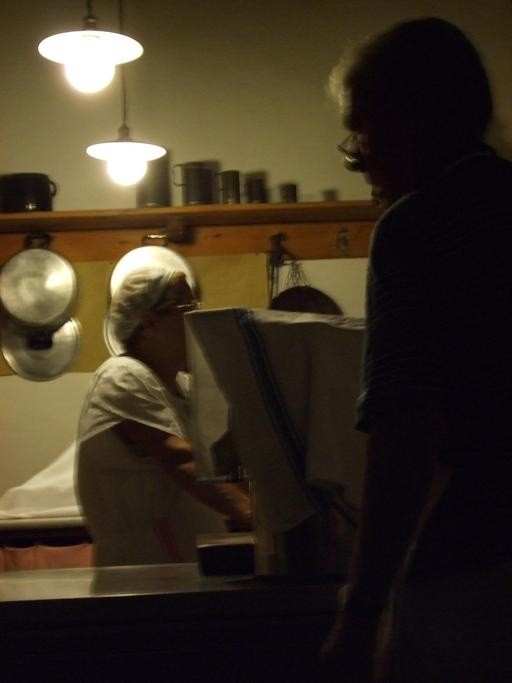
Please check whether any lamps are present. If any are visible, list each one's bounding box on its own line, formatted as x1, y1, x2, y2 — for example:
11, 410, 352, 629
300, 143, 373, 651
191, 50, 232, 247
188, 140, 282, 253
85, 1, 167, 188
37, 1, 146, 95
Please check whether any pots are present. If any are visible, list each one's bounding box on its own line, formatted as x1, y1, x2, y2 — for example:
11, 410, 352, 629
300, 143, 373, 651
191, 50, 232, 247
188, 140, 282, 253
2, 233, 81, 351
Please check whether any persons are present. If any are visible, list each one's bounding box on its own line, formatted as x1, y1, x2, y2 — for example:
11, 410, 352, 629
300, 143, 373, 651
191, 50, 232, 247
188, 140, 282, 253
73, 262, 254, 568
208, 283, 345, 535
315, 14, 512, 681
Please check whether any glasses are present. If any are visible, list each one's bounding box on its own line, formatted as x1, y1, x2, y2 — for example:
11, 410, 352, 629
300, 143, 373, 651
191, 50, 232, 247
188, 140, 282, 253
157, 300, 205, 316
337, 132, 371, 172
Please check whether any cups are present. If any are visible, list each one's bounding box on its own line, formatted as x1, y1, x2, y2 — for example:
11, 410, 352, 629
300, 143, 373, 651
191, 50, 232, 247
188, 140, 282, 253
135, 148, 170, 211
245, 172, 266, 205
214, 170, 240, 205
279, 183, 298, 204
1, 173, 57, 212
171, 162, 211, 206
